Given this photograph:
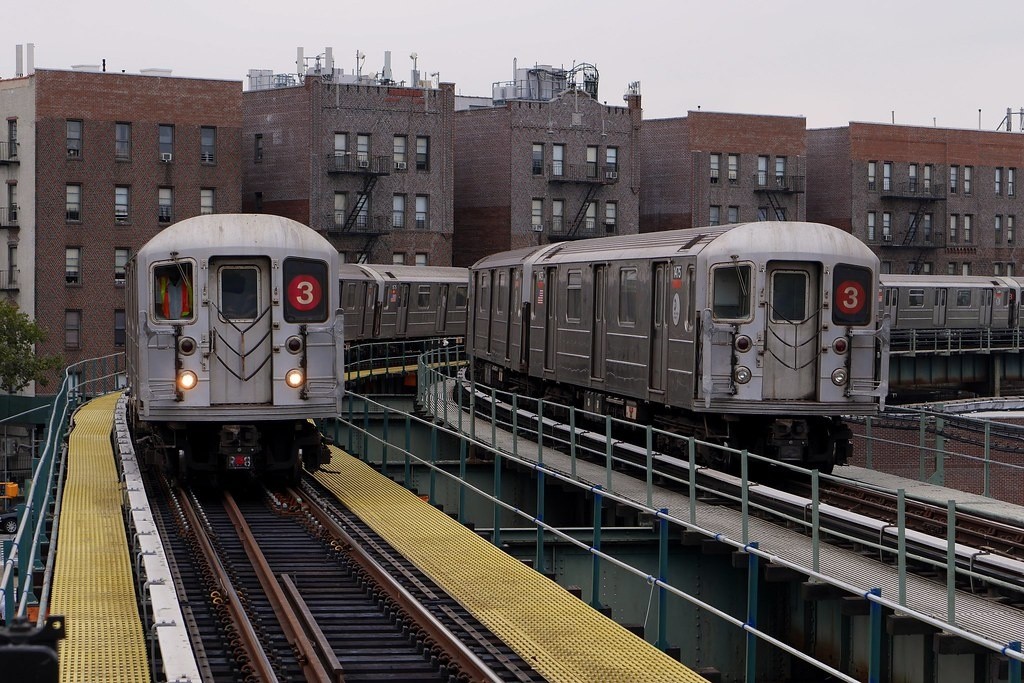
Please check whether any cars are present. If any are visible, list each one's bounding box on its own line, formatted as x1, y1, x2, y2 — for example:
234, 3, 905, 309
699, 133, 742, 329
0, 503, 54, 534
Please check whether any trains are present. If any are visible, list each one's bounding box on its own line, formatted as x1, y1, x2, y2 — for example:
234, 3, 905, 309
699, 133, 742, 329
124, 214, 477, 485
465, 221, 1023, 483
